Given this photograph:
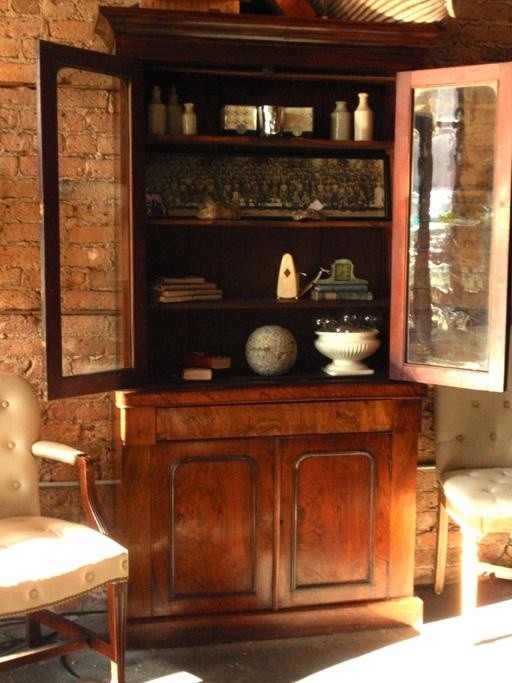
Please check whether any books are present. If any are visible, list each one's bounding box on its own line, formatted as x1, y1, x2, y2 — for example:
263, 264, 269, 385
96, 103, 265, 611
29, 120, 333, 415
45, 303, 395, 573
313, 283, 367, 291
152, 274, 218, 301
310, 288, 373, 301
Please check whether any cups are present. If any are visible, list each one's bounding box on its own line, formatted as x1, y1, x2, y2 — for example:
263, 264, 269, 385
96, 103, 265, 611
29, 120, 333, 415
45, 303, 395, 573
258, 105, 286, 134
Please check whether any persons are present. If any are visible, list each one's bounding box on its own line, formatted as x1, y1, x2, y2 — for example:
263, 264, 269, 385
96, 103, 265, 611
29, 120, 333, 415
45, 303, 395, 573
144, 153, 384, 212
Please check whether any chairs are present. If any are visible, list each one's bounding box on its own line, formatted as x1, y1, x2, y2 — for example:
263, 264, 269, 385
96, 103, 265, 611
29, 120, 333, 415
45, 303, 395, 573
0, 368, 131, 682
425, 312, 512, 611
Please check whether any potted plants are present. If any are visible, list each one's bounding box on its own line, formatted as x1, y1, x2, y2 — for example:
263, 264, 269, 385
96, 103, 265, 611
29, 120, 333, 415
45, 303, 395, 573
312, 311, 382, 377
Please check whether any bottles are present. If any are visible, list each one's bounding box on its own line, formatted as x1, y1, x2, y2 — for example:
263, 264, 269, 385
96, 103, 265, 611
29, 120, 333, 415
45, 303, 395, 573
167, 84, 185, 136
331, 101, 352, 140
180, 102, 198, 136
148, 84, 168, 136
352, 91, 374, 141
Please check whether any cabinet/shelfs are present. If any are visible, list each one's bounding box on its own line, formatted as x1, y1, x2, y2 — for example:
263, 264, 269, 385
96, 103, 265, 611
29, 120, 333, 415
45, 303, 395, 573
35, 1, 512, 649
